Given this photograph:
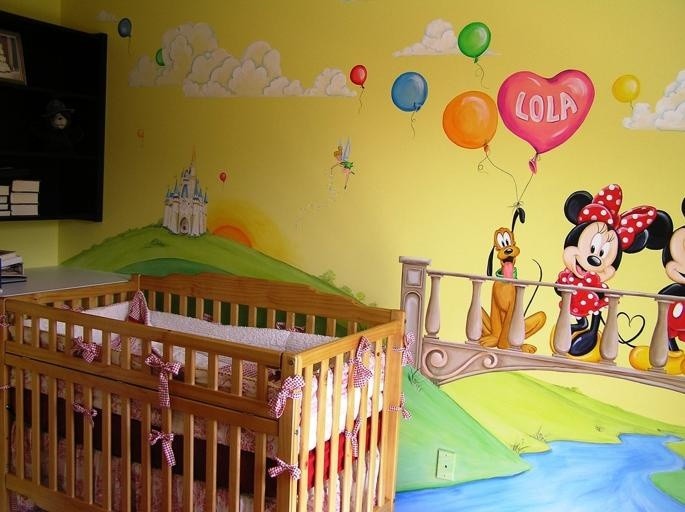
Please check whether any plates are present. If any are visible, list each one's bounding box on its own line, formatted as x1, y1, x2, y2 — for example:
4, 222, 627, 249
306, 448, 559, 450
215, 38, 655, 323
0, 10, 108, 223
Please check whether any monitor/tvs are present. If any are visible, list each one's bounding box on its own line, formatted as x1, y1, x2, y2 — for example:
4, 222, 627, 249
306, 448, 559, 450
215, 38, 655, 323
0, 204, 9, 211
0, 210, 10, 217
8, 192, 39, 205
0, 256, 21, 268
10, 180, 40, 193
9, 204, 39, 216
0, 195, 8, 204
0, 185, 10, 197
0, 249, 17, 261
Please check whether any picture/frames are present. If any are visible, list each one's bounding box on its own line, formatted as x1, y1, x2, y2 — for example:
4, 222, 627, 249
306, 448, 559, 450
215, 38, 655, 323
1, 264, 129, 298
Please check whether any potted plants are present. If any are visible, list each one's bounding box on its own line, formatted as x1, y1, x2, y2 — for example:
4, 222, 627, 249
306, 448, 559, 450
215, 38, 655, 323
0, 29, 27, 84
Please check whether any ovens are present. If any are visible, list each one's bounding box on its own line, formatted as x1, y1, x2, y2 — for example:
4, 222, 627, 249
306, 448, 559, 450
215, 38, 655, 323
1, 273, 406, 512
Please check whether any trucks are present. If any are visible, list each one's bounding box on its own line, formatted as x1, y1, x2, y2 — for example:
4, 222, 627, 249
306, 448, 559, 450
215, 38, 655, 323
349, 64, 368, 89
496, 68, 598, 177
389, 71, 429, 112
611, 74, 643, 107
457, 22, 493, 65
441, 91, 499, 150
